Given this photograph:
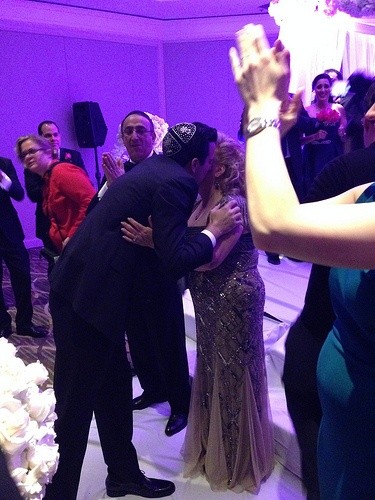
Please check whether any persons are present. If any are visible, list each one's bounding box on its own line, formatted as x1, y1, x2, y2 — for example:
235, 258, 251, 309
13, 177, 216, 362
227, 21, 375, 500
0, 156, 48, 338
14, 69, 375, 500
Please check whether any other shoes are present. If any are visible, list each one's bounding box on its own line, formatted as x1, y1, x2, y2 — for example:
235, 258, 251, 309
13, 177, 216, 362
268, 255, 280, 265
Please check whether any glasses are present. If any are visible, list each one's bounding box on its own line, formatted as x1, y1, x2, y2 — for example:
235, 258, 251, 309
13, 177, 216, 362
123, 128, 153, 135
22, 147, 47, 158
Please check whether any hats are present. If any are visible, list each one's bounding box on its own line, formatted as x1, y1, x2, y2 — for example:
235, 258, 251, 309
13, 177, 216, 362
162, 122, 199, 159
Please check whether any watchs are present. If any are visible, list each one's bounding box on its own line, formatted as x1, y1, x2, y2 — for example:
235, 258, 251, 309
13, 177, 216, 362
246, 117, 281, 140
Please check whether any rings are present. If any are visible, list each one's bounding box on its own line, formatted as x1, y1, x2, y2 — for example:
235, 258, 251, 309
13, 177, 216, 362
110, 167, 113, 170
133, 236, 137, 242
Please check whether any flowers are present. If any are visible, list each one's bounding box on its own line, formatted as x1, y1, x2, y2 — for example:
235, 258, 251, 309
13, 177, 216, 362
112, 112, 170, 163
315, 108, 341, 142
0, 336, 60, 500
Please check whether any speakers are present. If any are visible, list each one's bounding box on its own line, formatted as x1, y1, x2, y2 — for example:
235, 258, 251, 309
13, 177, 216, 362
72, 101, 109, 148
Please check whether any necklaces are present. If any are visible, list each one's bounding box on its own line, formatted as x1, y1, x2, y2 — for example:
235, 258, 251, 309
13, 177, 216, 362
194, 191, 215, 220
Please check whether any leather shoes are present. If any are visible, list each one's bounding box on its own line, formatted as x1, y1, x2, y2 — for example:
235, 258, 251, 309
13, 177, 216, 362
0, 327, 12, 338
165, 408, 189, 436
17, 326, 49, 338
131, 391, 158, 410
106, 469, 176, 498
